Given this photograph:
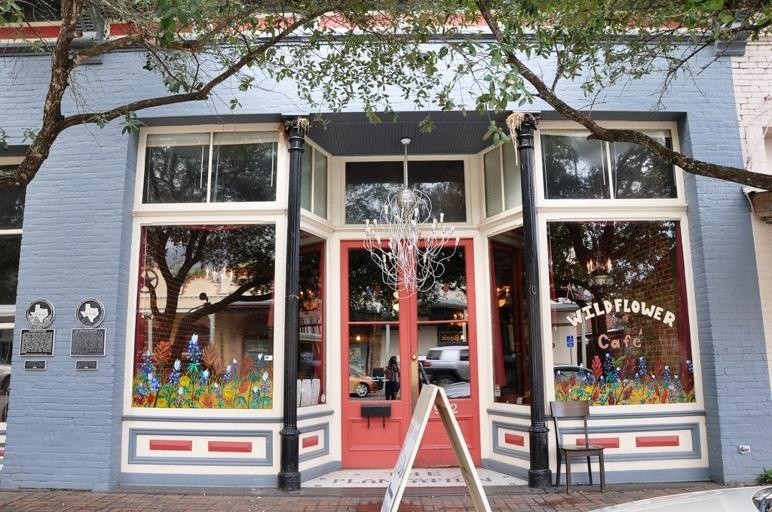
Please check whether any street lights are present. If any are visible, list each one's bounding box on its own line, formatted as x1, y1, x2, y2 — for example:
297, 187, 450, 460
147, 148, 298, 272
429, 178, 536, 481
590, 266, 618, 384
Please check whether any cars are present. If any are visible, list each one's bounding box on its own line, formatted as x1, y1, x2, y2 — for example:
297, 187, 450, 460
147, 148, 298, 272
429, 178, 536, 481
553, 364, 596, 386
348, 364, 377, 398
417, 345, 470, 386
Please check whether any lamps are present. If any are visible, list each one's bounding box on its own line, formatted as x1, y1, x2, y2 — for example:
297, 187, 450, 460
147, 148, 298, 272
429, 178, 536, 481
363, 139, 460, 300
452, 312, 469, 331
586, 241, 612, 273
301, 290, 319, 309
496, 286, 510, 307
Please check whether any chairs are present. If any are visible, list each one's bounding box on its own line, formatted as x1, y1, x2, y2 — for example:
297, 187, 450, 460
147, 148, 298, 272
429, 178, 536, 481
550, 400, 606, 495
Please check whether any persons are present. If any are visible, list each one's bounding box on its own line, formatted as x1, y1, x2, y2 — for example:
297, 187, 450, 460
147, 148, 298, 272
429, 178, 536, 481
384, 356, 400, 399
